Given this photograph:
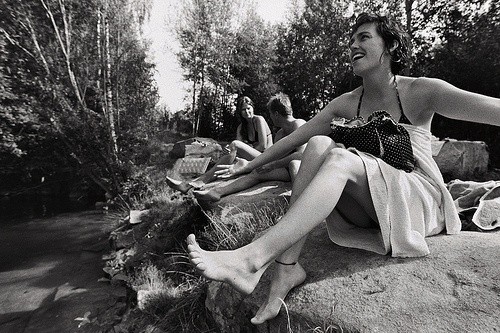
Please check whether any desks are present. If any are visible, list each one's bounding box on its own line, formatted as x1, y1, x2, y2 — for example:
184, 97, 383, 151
432, 141, 489, 177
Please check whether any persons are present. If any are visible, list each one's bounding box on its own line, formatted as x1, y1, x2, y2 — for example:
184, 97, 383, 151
192, 93, 309, 202
165, 96, 273, 194
185, 11, 499, 324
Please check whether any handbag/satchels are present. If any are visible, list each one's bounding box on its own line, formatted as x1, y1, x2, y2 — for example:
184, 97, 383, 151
329, 108, 415, 173
